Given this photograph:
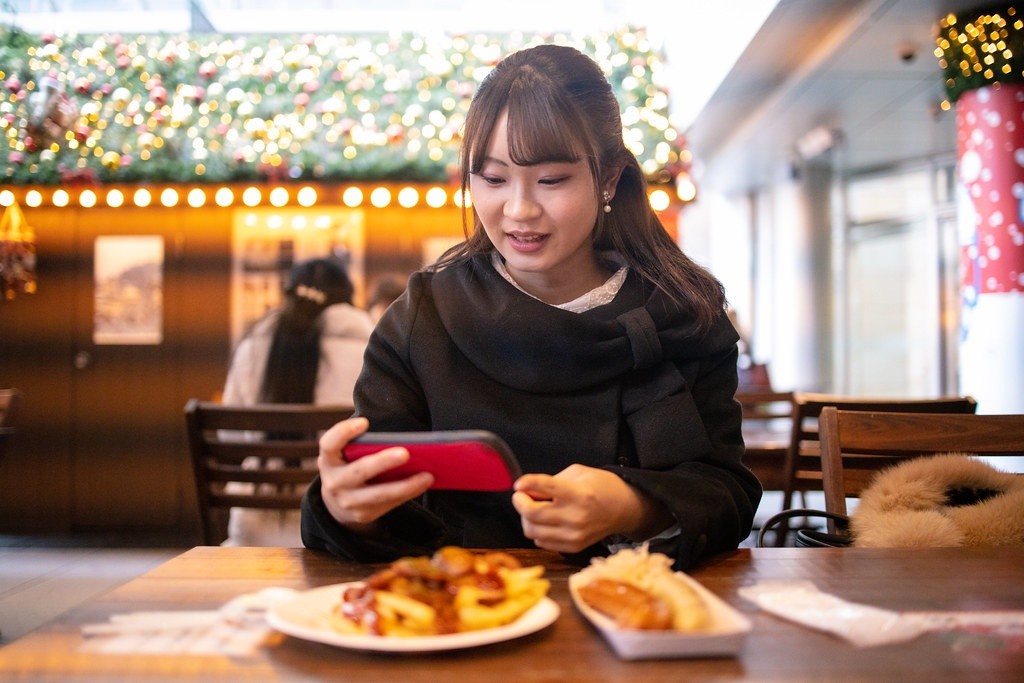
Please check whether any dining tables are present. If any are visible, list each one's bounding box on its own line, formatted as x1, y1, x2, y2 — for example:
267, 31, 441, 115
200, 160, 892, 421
1, 546, 1024, 683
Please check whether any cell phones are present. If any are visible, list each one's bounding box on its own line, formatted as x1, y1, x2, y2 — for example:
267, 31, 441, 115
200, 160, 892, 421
340, 428, 520, 492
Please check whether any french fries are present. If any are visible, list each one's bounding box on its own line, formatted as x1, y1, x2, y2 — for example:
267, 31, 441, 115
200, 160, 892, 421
334, 564, 550, 638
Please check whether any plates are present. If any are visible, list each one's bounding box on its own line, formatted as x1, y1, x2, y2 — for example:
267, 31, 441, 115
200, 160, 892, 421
264, 583, 562, 653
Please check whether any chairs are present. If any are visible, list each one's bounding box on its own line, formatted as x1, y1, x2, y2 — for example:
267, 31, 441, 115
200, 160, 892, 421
182, 398, 357, 546
735, 390, 1024, 538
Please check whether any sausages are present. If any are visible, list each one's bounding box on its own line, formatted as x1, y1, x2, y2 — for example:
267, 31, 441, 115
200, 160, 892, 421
641, 571, 711, 631
578, 575, 674, 629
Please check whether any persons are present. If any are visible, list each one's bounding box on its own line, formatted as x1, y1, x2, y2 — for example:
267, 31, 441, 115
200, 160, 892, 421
301, 44, 764, 563
369, 274, 407, 323
216, 257, 373, 546
